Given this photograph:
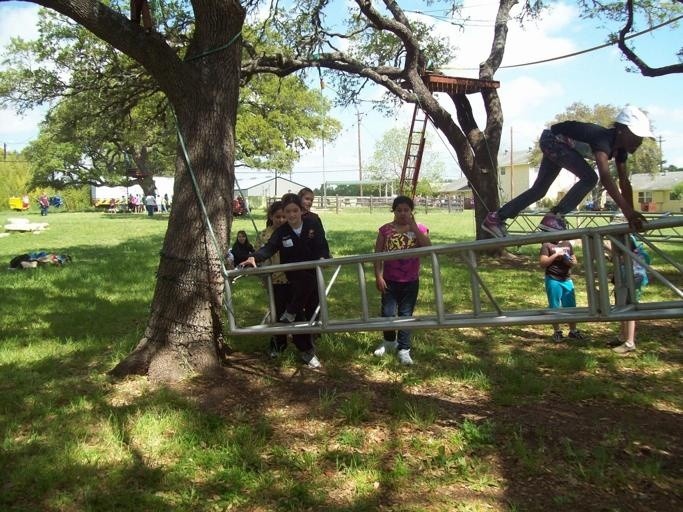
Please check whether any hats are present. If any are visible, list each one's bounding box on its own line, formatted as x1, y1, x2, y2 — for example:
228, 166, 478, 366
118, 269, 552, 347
614, 106, 657, 141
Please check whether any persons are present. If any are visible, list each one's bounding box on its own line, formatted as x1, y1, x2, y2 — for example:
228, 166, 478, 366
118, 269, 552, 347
227, 187, 332, 370
480, 108, 655, 239
606, 233, 649, 353
373, 196, 432, 365
109, 192, 169, 216
39, 191, 49, 216
539, 240, 586, 343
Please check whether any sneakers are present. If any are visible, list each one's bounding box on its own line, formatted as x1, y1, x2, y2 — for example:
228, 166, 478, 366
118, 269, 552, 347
374, 340, 413, 365
539, 211, 575, 231
606, 337, 636, 353
481, 212, 511, 239
302, 351, 320, 369
552, 330, 584, 342
280, 308, 296, 323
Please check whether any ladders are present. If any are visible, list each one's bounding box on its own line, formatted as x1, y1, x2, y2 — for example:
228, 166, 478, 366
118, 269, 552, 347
399, 91, 433, 199
222, 216, 683, 335
